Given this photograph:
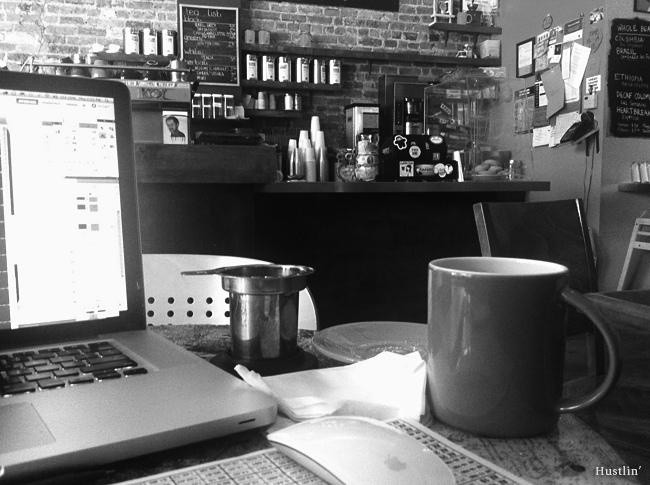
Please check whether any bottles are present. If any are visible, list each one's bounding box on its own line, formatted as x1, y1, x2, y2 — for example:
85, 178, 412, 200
328, 59, 341, 85
142, 28, 158, 57
161, 29, 177, 57
262, 55, 276, 83
313, 58, 327, 84
245, 53, 259, 82
296, 57, 309, 83
278, 56, 292, 83
122, 27, 140, 56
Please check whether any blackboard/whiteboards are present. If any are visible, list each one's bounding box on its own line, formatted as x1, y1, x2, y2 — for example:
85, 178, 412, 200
179, 4, 240, 87
607, 18, 650, 137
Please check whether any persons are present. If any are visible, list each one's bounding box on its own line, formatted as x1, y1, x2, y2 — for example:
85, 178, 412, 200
166, 117, 185, 139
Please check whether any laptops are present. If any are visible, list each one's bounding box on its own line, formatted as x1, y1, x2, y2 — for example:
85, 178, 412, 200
0, 69, 279, 478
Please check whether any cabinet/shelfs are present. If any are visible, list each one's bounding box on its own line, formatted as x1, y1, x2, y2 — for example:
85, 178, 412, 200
34, 18, 514, 119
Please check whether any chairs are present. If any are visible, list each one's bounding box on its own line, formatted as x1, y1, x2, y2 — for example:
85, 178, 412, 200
140, 253, 318, 334
473, 196, 601, 337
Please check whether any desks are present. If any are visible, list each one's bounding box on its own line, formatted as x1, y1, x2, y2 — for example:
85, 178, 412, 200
0, 334, 650, 485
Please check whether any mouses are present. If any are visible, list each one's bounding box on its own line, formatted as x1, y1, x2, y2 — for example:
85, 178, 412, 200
265, 415, 458, 485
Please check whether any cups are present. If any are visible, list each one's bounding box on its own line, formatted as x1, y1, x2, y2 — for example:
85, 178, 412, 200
242, 91, 302, 111
426, 255, 621, 439
285, 113, 332, 184
180, 261, 314, 360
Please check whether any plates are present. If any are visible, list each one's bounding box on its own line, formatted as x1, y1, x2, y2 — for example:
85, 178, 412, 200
309, 318, 429, 366
